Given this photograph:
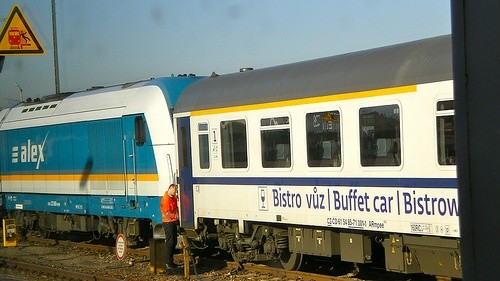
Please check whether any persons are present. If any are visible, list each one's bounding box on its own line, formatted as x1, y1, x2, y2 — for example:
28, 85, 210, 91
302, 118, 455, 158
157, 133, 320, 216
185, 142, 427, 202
161, 183, 179, 270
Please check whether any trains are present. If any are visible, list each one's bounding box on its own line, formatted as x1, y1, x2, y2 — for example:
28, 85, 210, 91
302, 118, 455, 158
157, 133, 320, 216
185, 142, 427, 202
0, 33, 462, 281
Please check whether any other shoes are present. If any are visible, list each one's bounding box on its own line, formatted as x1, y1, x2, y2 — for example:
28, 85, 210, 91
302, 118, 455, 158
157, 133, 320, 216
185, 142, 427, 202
166, 263, 179, 269
